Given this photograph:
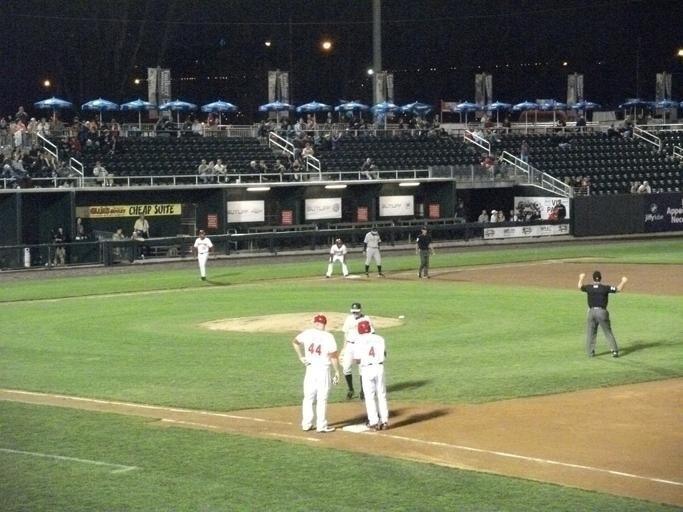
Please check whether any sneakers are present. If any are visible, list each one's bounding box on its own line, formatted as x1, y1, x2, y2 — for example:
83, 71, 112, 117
321, 427, 336, 432
366, 422, 379, 431
380, 423, 389, 430
347, 389, 354, 399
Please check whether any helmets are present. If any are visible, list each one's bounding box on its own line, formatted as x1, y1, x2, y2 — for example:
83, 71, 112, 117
350, 303, 360, 312
314, 315, 326, 324
358, 322, 370, 334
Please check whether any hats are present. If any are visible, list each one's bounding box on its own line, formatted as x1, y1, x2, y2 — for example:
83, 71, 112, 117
592, 271, 600, 281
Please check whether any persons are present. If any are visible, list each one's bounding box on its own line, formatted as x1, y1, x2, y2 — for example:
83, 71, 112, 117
1, 96, 681, 280
578, 271, 628, 357
292, 316, 340, 432
342, 304, 375, 399
353, 321, 389, 430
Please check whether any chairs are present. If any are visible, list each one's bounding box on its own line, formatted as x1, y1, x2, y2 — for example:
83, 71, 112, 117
40, 127, 683, 198
